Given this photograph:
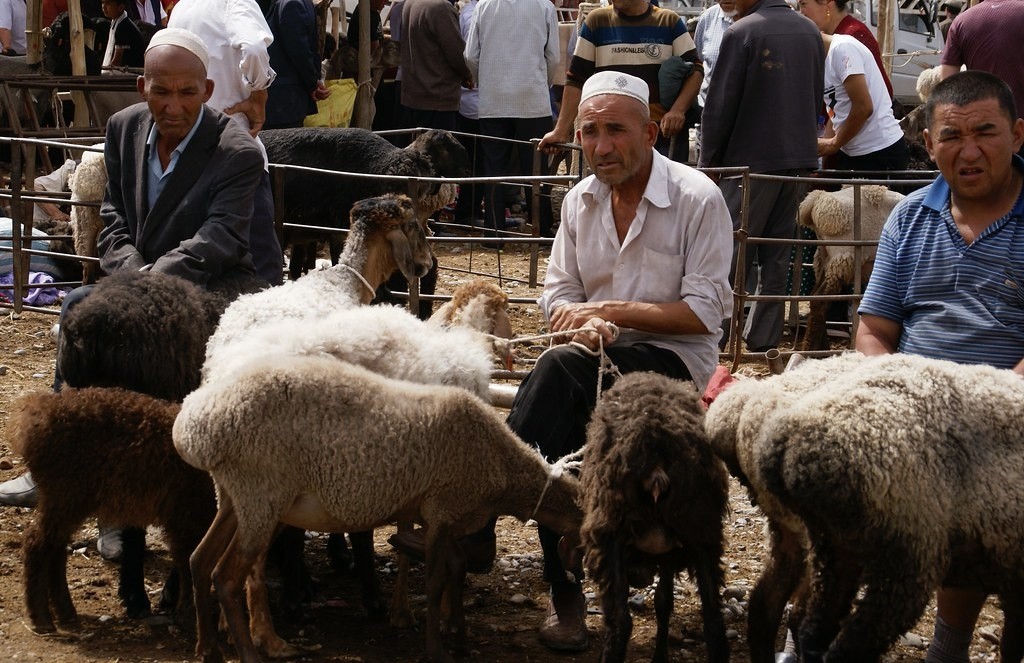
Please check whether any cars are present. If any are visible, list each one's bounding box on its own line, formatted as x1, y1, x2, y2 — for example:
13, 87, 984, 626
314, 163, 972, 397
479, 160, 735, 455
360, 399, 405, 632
848, 0, 946, 120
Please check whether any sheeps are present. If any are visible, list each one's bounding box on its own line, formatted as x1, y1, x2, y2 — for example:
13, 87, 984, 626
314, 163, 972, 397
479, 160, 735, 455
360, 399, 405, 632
170, 354, 589, 663
796, 185, 907, 353
580, 371, 731, 663
6, 386, 219, 636
702, 352, 1024, 663
44, 126, 532, 627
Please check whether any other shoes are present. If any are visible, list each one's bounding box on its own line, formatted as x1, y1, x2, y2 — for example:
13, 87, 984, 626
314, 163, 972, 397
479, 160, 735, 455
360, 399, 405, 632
480, 244, 503, 250
97, 524, 123, 558
1, 472, 44, 507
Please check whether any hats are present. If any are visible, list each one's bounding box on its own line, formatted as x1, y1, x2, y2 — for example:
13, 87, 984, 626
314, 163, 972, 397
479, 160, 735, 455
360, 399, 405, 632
145, 28, 211, 77
579, 71, 650, 110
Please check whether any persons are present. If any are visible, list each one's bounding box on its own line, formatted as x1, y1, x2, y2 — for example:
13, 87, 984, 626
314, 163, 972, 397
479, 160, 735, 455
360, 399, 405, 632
0, 0, 909, 354
0, 28, 285, 560
941, 0, 1024, 161
771, 71, 1024, 663
385, 70, 734, 653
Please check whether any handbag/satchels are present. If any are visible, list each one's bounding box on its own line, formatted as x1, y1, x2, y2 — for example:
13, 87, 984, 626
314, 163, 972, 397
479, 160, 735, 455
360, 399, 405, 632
330, 44, 359, 79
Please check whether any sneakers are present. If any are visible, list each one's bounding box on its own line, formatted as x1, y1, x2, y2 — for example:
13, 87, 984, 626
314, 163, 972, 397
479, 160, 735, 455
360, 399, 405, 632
541, 594, 590, 652
